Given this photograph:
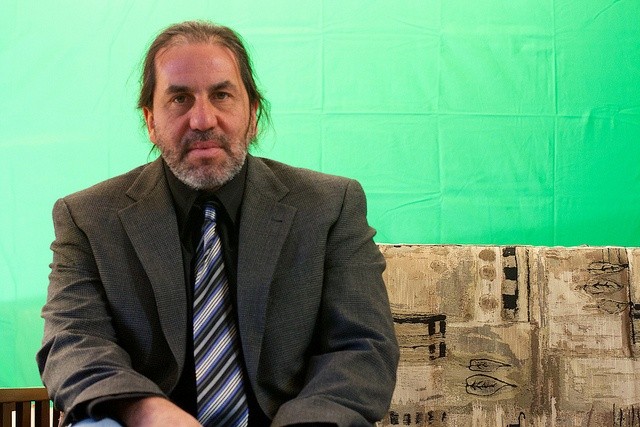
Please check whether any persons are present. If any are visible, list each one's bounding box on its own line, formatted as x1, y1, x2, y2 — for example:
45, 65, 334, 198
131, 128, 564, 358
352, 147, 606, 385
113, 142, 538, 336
34, 18, 400, 426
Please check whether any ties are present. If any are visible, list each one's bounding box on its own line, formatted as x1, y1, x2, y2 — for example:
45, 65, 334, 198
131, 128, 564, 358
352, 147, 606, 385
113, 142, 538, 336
191, 201, 249, 427
